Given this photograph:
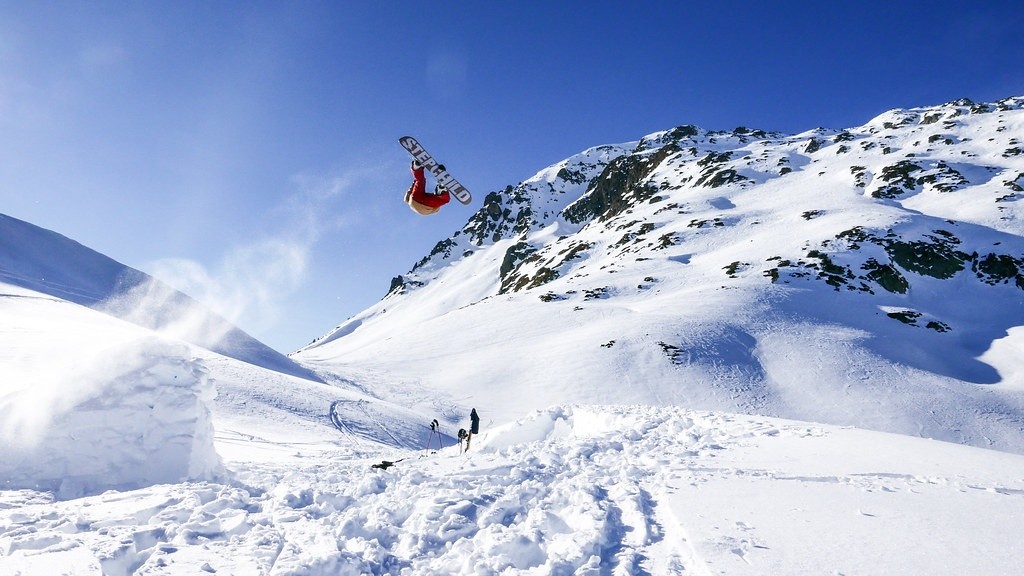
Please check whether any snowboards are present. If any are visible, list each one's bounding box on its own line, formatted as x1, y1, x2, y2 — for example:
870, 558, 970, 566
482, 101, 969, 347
397, 135, 472, 206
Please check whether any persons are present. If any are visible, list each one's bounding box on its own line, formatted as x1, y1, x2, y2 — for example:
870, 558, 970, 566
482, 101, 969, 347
404, 159, 451, 216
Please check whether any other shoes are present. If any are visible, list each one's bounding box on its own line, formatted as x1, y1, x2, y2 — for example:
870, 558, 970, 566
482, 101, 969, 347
437, 185, 447, 196
413, 159, 422, 170
